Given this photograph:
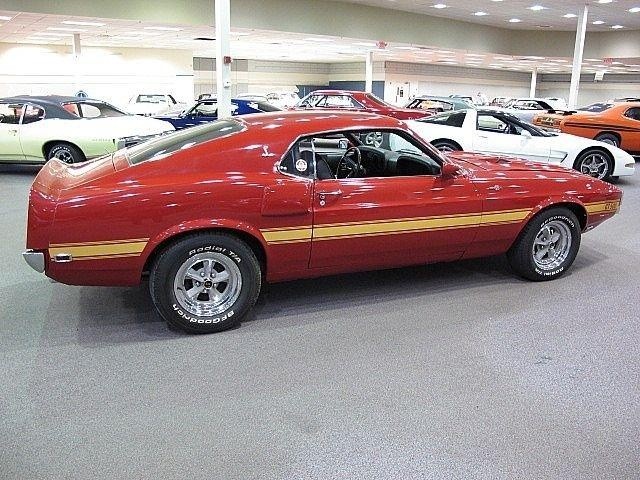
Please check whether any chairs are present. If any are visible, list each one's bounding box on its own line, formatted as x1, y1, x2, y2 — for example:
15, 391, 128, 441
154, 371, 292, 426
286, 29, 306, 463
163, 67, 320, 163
300, 150, 335, 179
